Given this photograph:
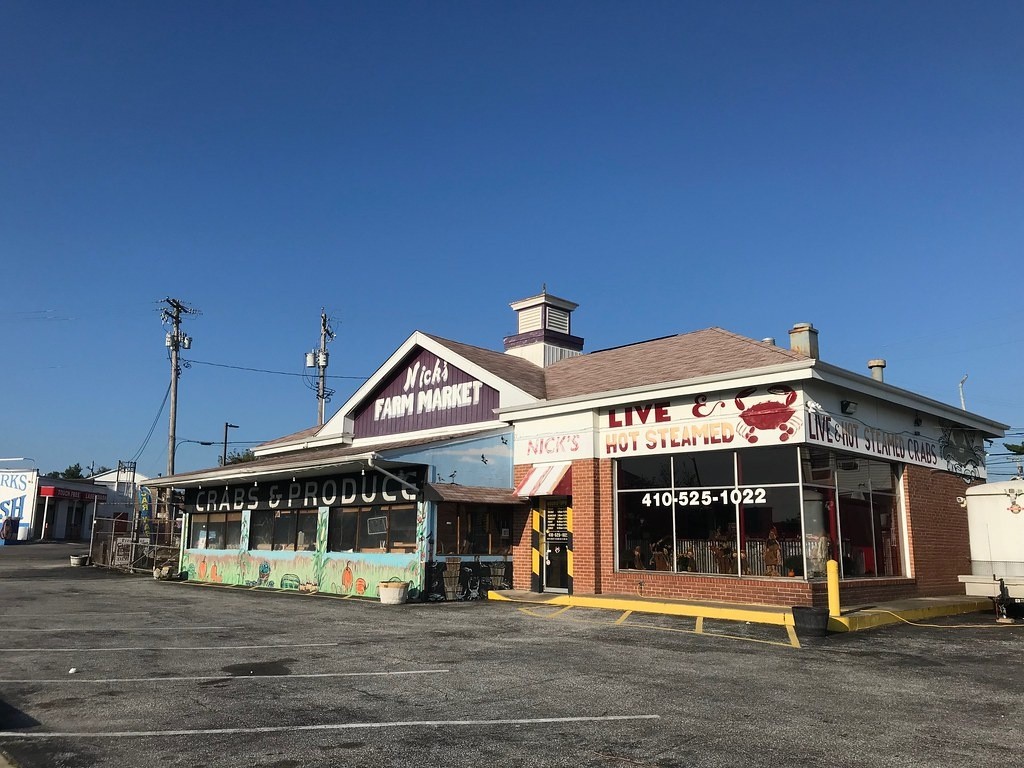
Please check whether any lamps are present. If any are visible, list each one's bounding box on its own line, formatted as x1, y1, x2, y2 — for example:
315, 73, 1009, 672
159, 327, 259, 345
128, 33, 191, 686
841, 400, 858, 415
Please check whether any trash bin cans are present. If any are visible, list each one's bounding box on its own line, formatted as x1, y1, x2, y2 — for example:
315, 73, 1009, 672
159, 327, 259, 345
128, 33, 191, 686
792, 605, 830, 637
70, 554, 89, 566
379, 581, 410, 605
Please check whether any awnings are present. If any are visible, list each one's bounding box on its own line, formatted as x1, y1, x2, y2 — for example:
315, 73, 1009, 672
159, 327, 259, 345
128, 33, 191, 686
515, 462, 572, 496
40, 485, 107, 501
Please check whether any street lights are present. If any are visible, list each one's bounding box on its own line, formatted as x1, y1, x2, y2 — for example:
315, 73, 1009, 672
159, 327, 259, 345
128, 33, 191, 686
167, 439, 212, 475
222, 422, 240, 465
